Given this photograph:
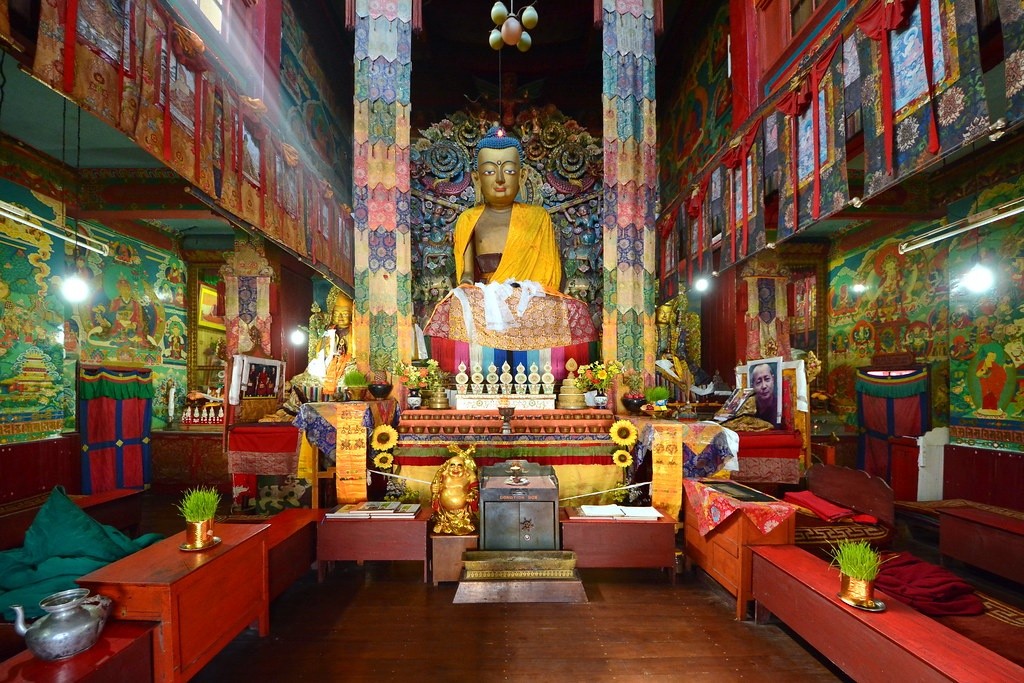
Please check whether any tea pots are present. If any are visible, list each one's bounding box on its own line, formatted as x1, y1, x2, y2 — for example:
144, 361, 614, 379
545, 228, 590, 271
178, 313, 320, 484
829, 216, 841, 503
8, 588, 111, 661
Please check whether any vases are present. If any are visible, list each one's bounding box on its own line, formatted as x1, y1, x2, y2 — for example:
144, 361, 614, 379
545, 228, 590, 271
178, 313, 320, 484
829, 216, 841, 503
593, 389, 609, 409
405, 389, 424, 411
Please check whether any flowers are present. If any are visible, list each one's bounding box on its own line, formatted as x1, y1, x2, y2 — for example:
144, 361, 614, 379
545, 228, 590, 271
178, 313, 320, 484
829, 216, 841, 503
574, 358, 624, 397
393, 358, 446, 390
371, 421, 419, 505
607, 419, 641, 505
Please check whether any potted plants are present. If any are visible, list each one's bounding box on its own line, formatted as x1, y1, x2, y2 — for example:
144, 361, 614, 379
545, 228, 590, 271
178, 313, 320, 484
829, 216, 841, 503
819, 537, 902, 612
171, 483, 223, 551
647, 386, 670, 417
344, 368, 371, 402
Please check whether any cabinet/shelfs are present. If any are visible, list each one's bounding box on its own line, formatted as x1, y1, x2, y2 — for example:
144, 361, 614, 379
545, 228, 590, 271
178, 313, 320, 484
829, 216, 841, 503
558, 509, 679, 587
74, 522, 271, 683
0, 621, 161, 682
935, 506, 1024, 584
314, 505, 435, 583
251, 508, 327, 632
746, 543, 1024, 683
475, 458, 560, 552
682, 477, 811, 621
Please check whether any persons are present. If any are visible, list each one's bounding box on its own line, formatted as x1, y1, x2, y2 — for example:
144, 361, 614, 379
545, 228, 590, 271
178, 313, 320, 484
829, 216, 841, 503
431, 456, 478, 536
750, 362, 777, 422
305, 290, 355, 392
246, 365, 276, 397
656, 303, 695, 391
425, 127, 598, 389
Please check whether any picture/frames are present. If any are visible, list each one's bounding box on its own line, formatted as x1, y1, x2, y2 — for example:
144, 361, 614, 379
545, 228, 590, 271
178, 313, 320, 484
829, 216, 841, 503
241, 355, 280, 399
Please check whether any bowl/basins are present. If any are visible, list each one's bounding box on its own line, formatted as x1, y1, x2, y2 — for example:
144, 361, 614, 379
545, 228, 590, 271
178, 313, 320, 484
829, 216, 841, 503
398, 412, 504, 434
640, 403, 663, 419
620, 397, 647, 414
510, 413, 613, 433
368, 384, 394, 400
